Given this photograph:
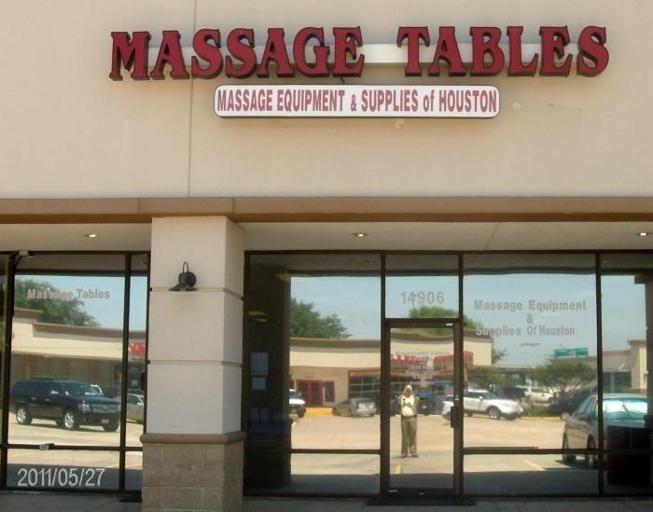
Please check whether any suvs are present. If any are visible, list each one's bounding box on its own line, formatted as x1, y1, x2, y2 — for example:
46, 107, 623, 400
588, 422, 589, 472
288, 388, 306, 419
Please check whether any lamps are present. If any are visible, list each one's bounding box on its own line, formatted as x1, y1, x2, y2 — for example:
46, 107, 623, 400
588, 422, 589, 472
164, 259, 198, 295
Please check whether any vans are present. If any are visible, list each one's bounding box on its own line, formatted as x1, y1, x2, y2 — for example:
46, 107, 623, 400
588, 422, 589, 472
7, 376, 119, 432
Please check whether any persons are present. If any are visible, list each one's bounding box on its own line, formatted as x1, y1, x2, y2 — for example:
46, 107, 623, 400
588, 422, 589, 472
394, 385, 420, 458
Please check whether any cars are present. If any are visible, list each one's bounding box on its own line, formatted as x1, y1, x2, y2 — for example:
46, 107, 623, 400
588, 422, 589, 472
82, 384, 104, 397
389, 384, 599, 423
331, 395, 377, 418
109, 392, 144, 425
559, 392, 647, 471
